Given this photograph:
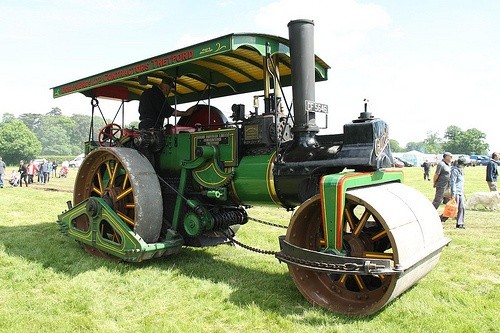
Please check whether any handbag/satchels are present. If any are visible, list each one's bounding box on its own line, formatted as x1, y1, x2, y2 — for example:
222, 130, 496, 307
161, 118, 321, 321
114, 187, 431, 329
443, 198, 458, 217
443, 182, 452, 197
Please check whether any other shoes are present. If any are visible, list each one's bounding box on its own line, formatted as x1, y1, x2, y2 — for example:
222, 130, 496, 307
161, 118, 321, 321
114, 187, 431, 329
456, 224, 465, 229
443, 198, 450, 204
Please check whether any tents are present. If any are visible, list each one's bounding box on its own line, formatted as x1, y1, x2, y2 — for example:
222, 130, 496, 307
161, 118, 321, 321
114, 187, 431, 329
409, 150, 436, 167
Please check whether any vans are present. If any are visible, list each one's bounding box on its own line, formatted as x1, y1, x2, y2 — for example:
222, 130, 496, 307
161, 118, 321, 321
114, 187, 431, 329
68, 153, 85, 169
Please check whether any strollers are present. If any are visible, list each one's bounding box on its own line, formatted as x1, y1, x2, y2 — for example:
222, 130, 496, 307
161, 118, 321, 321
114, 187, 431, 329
9, 170, 22, 188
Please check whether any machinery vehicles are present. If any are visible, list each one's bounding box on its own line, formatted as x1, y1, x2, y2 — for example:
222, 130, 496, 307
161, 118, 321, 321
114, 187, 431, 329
55, 19, 452, 319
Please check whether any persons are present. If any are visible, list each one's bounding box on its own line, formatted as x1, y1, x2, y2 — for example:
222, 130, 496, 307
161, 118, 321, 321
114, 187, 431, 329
138, 78, 199, 130
439, 158, 468, 229
420, 152, 500, 209
0, 157, 69, 188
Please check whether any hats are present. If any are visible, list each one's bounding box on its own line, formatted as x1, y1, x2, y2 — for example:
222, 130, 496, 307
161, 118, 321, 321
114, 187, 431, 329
163, 78, 173, 87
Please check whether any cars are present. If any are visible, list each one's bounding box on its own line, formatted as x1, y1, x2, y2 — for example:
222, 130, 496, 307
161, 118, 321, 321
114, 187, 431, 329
457, 154, 489, 167
393, 156, 414, 168
